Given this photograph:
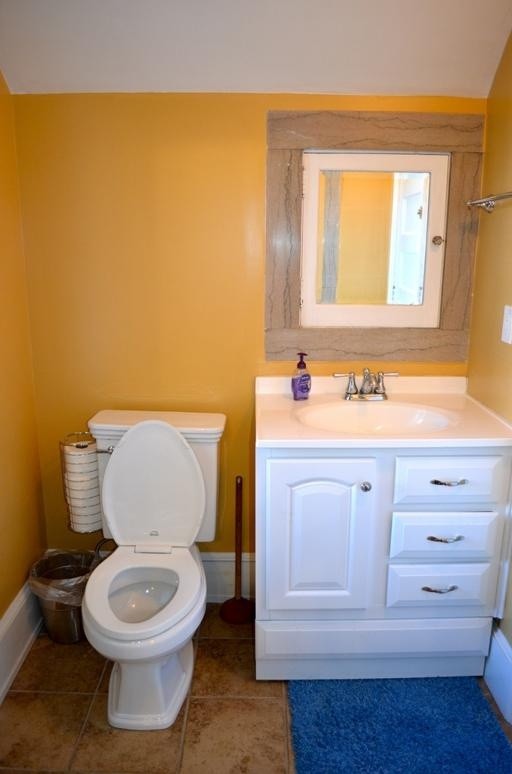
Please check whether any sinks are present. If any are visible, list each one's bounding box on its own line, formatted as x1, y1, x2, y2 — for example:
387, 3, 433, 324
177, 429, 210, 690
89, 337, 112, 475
303, 405, 451, 432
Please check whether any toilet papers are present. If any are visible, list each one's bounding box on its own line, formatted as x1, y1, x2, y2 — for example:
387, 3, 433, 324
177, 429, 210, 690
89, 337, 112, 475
64, 440, 103, 534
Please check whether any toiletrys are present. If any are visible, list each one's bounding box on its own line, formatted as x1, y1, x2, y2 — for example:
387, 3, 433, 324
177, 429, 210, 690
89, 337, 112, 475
290, 352, 312, 401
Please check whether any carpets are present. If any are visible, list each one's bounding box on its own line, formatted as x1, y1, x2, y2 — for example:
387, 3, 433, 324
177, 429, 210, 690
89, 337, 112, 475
289, 678, 512, 774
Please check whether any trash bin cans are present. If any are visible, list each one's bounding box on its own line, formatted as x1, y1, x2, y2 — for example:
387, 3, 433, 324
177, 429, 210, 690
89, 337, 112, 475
31, 551, 102, 644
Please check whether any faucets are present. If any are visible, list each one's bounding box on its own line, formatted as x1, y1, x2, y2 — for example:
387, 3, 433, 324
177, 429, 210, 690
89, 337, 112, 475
359, 366, 374, 394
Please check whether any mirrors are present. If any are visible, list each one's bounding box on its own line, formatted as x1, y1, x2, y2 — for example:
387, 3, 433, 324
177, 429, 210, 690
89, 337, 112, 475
300, 152, 451, 328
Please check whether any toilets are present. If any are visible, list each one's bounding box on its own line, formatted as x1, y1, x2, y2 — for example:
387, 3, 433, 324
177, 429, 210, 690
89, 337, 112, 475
80, 408, 228, 733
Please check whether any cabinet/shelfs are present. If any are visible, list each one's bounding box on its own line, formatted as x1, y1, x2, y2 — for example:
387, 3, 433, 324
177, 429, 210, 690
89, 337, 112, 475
261, 454, 383, 620
385, 456, 510, 616
